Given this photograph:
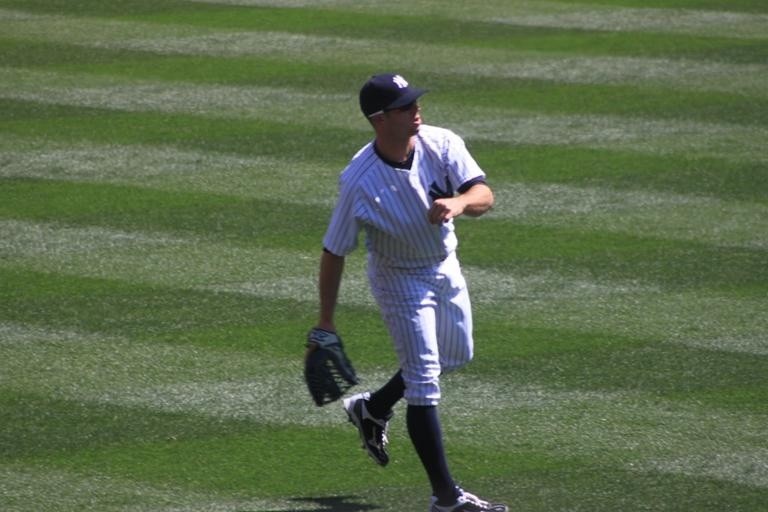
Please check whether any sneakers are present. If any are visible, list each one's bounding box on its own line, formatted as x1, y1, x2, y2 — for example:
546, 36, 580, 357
342, 391, 397, 467
428, 486, 511, 512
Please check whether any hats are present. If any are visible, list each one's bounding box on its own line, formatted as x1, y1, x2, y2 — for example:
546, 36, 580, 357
359, 72, 431, 118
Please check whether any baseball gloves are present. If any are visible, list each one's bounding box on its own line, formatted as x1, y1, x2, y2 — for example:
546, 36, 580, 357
305, 326, 360, 406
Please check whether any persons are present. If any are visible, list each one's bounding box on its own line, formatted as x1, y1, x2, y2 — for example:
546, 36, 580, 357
316, 73, 508, 511
